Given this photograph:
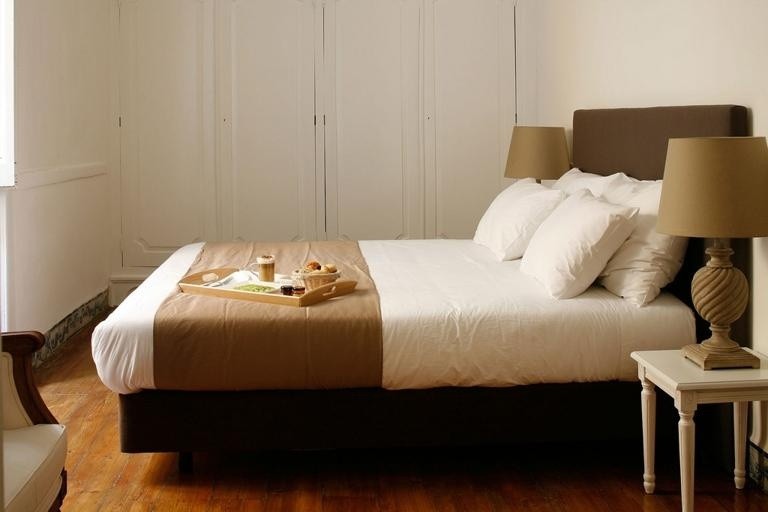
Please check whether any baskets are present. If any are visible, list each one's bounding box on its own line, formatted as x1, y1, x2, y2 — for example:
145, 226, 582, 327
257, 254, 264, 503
293, 269, 341, 294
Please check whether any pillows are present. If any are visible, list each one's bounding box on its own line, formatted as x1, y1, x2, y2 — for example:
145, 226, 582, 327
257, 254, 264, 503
474, 169, 688, 308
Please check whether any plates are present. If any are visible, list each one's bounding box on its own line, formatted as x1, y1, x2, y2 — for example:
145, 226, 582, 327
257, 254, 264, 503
234, 283, 280, 294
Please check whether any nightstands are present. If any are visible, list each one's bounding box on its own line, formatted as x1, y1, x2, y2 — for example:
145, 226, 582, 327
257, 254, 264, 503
630, 345, 768, 512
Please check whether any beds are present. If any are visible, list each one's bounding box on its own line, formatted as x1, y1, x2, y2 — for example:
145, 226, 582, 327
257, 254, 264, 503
90, 104, 752, 475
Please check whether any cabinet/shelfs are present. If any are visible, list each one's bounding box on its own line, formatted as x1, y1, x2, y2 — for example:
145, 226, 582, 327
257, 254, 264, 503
106, 0, 538, 309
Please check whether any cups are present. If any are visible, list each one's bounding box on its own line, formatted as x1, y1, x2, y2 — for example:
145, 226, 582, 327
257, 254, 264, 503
250, 256, 275, 283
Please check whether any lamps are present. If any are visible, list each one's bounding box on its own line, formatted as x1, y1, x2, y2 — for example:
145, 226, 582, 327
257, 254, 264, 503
658, 135, 768, 370
504, 126, 572, 184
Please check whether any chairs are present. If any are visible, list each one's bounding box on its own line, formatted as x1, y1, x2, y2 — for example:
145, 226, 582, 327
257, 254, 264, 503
0, 329, 68, 512
